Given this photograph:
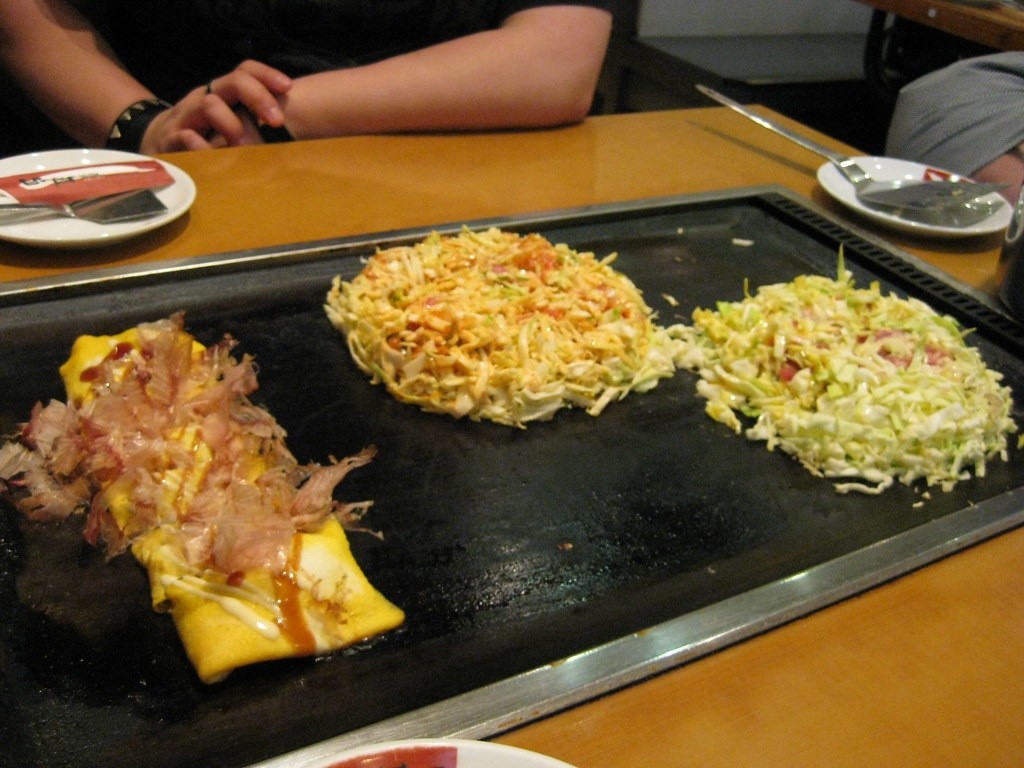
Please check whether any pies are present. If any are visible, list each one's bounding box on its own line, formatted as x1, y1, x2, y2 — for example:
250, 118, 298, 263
60, 325, 407, 685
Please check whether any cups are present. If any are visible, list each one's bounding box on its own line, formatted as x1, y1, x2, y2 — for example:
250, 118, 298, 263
993, 178, 1024, 319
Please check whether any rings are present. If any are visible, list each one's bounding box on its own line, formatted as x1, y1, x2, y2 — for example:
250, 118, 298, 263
206, 80, 214, 95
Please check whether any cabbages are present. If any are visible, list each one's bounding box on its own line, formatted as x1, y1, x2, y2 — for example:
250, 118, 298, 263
327, 228, 678, 430
676, 241, 1018, 493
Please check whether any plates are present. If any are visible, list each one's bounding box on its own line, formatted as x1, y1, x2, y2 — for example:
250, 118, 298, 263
0, 148, 196, 252
816, 158, 1013, 240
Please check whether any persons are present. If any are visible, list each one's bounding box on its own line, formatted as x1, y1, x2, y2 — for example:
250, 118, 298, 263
0, 0, 623, 155
884, 50, 1024, 208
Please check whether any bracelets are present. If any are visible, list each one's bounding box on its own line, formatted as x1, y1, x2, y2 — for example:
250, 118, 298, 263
108, 99, 172, 153
243, 103, 296, 144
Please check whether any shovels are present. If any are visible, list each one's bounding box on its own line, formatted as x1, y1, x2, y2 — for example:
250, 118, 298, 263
695, 85, 1013, 212
0, 190, 168, 224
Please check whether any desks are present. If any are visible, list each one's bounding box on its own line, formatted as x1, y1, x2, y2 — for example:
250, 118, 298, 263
0, 103, 1024, 768
